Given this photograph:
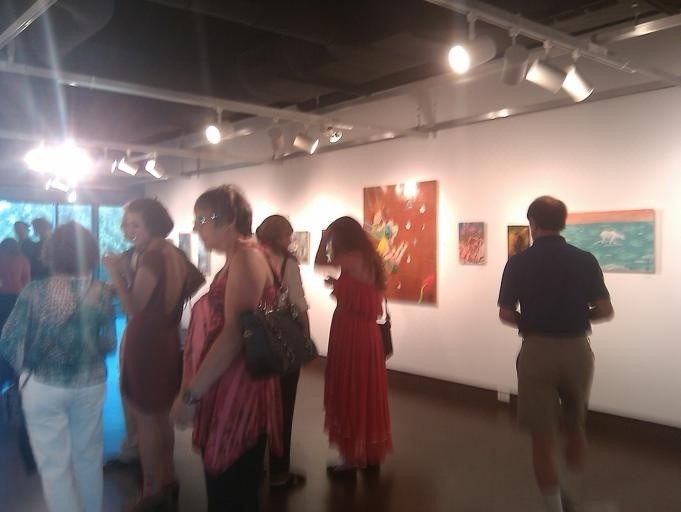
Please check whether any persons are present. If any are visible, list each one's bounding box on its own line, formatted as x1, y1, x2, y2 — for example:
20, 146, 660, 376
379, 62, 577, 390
497, 195, 615, 512
0, 216, 116, 512
313, 216, 395, 479
102, 182, 309, 512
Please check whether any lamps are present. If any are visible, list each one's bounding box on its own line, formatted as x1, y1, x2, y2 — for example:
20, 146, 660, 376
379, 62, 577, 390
447, 11, 596, 104
205, 107, 343, 159
109, 149, 166, 180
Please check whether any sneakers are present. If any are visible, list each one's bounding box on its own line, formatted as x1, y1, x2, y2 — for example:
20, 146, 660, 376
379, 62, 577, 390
268, 459, 384, 493
102, 456, 140, 474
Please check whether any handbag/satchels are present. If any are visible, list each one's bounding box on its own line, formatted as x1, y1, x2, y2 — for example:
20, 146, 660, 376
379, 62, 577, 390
375, 316, 396, 359
236, 246, 320, 382
4, 384, 25, 434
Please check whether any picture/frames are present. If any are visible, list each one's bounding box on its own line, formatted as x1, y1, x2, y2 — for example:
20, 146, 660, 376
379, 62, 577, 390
507, 226, 530, 258
